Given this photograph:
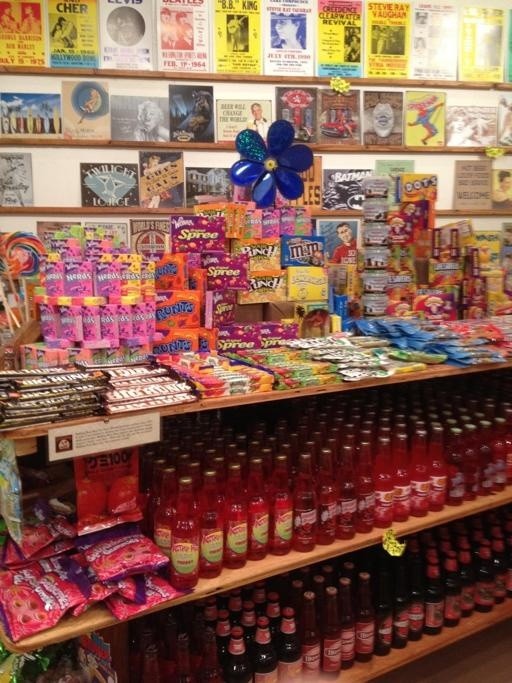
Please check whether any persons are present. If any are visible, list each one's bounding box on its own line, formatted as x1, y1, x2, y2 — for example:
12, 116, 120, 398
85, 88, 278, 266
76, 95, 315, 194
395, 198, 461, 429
307, 310, 324, 338
331, 224, 358, 266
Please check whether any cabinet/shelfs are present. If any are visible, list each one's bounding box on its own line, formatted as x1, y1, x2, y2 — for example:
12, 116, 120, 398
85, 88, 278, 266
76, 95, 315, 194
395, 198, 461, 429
0, 353, 511, 683
0, 64, 512, 218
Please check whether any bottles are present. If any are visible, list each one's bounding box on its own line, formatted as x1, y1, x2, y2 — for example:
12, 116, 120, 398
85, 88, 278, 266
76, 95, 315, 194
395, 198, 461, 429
129, 387, 511, 683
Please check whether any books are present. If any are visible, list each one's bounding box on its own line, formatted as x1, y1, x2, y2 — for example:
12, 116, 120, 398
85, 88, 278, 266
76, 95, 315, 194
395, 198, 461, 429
1, 2, 510, 211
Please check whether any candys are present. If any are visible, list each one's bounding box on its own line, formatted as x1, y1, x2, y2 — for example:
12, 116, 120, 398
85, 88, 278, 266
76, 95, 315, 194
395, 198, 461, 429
0, 229, 46, 332
